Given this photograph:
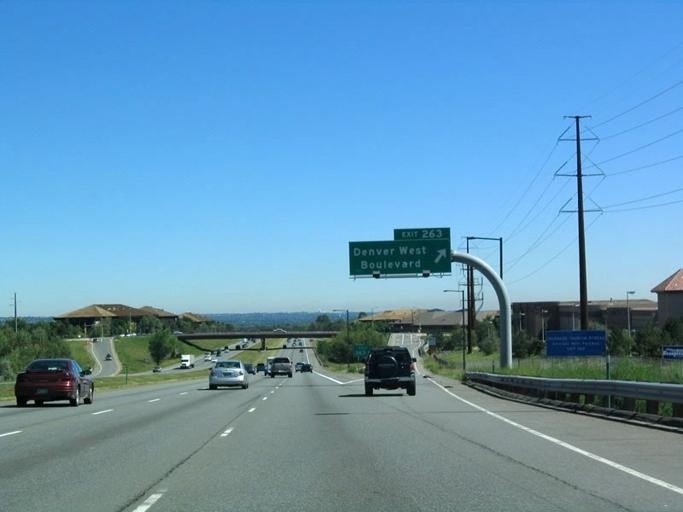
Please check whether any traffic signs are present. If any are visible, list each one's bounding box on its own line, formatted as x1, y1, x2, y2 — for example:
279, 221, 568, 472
347, 238, 452, 276
545, 330, 607, 358
392, 228, 450, 239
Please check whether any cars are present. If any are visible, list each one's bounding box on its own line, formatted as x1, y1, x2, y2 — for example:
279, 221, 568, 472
92, 338, 100, 343
291, 337, 305, 352
105, 352, 113, 361
13, 357, 94, 405
152, 367, 163, 373
207, 360, 251, 389
172, 331, 183, 335
272, 328, 288, 334
242, 361, 315, 375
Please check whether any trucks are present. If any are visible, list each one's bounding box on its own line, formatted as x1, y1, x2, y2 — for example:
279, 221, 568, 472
200, 337, 257, 363
178, 354, 196, 369
266, 356, 276, 374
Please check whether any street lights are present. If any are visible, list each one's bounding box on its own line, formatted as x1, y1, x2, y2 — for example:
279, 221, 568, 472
626, 289, 637, 356
518, 311, 525, 332
541, 308, 549, 347
573, 302, 580, 332
331, 309, 351, 370
443, 289, 467, 373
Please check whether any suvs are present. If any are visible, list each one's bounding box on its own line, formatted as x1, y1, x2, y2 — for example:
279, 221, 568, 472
269, 357, 294, 378
360, 345, 421, 397
